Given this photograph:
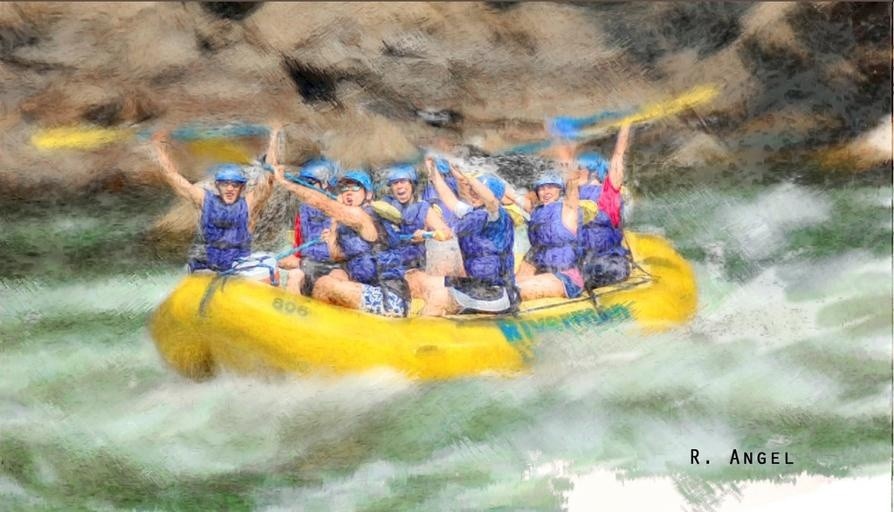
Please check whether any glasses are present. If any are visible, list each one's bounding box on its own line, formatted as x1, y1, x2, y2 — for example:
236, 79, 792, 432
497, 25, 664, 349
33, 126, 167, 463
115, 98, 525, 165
340, 184, 361, 191
307, 180, 318, 186
218, 181, 240, 188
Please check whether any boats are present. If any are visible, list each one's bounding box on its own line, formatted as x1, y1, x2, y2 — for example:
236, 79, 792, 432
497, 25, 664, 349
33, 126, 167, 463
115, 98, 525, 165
140, 219, 701, 393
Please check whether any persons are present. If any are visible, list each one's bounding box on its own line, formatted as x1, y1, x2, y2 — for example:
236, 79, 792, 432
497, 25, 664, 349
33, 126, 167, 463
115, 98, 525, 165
411, 151, 521, 319
473, 141, 580, 298
275, 155, 346, 270
557, 117, 647, 286
149, 118, 284, 291
366, 166, 454, 270
263, 162, 410, 317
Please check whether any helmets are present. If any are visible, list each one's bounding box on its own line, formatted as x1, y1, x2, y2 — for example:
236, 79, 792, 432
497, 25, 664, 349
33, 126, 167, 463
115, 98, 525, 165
473, 174, 506, 200
434, 159, 450, 173
574, 152, 608, 182
533, 171, 564, 191
386, 164, 418, 187
214, 162, 247, 184
300, 158, 337, 187
337, 169, 373, 191
